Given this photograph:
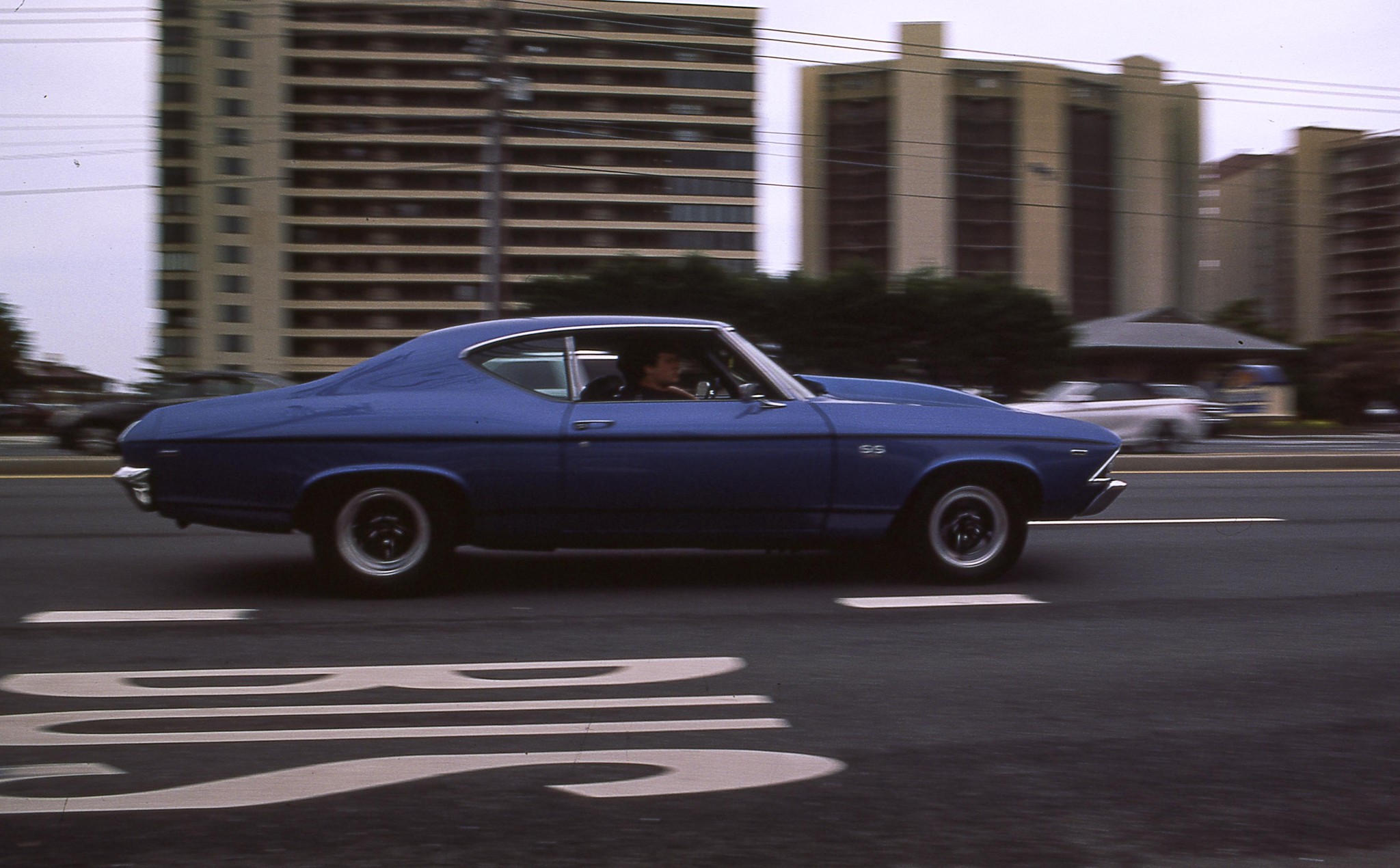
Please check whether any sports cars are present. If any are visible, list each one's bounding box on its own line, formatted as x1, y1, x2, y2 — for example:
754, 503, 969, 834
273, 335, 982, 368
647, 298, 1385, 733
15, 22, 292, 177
111, 316, 1130, 602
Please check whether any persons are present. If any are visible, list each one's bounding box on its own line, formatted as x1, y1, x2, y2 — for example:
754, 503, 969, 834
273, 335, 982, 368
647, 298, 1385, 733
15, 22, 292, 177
635, 341, 699, 400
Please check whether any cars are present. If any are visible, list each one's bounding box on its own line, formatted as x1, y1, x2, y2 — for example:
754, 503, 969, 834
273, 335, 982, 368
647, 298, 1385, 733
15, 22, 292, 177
1143, 383, 1231, 437
1004, 380, 1204, 455
